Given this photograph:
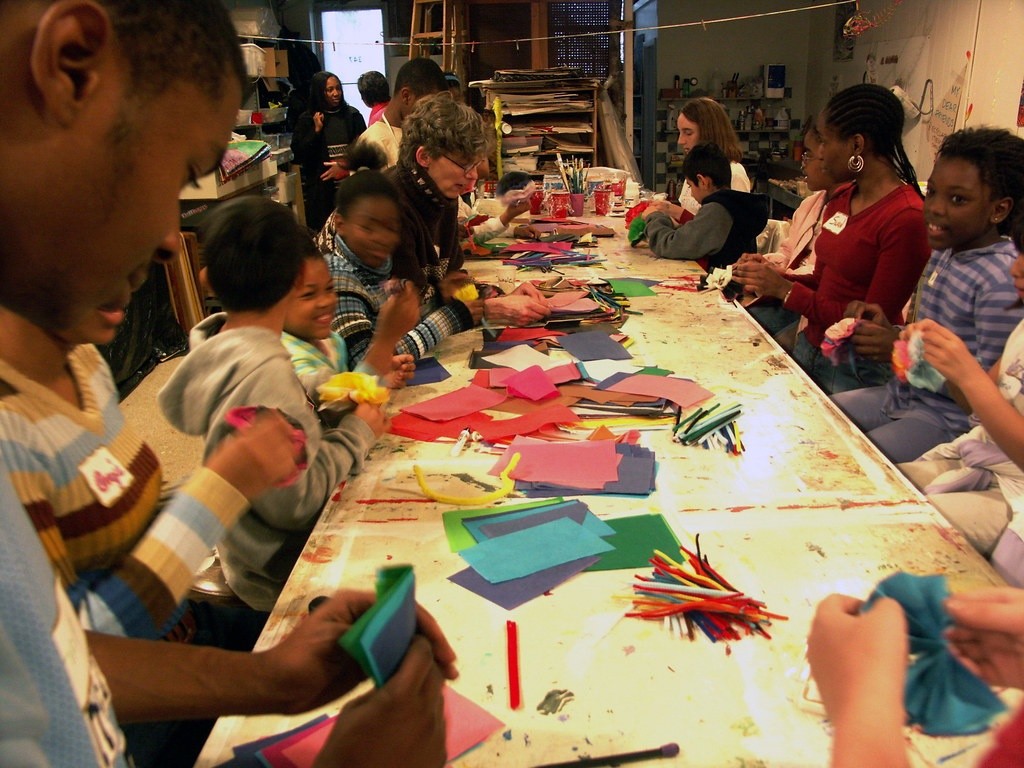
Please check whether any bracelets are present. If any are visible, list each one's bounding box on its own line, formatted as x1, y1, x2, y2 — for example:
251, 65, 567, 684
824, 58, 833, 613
784, 291, 791, 303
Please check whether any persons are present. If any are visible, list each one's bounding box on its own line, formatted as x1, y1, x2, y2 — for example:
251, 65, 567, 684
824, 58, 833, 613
311, 143, 483, 370
0, 272, 309, 768
731, 85, 932, 395
806, 587, 1024, 768
291, 70, 392, 233
642, 141, 770, 298
894, 206, 1024, 590
744, 127, 850, 335
828, 127, 1023, 463
383, 94, 551, 327
0, 0, 459, 768
280, 244, 421, 413
159, 196, 386, 612
652, 97, 750, 269
358, 57, 541, 245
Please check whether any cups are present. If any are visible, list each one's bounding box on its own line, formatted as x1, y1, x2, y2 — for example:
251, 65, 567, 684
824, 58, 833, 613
529, 190, 545, 215
495, 265, 518, 293
571, 194, 585, 217
549, 192, 571, 218
594, 190, 610, 216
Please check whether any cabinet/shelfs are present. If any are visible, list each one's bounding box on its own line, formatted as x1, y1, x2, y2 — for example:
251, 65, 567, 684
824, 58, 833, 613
483, 85, 600, 168
659, 96, 786, 175
179, 36, 298, 232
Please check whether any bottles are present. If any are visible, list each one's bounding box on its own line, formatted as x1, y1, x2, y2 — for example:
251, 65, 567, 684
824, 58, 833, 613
738, 111, 746, 130
683, 78, 690, 97
625, 182, 640, 208
674, 75, 680, 98
774, 106, 789, 129
609, 183, 624, 212
753, 106, 765, 131
793, 133, 801, 162
765, 105, 774, 130
708, 72, 720, 97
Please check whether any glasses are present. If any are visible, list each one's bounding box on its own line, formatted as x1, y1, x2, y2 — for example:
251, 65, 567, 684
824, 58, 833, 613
800, 150, 819, 161
434, 149, 484, 173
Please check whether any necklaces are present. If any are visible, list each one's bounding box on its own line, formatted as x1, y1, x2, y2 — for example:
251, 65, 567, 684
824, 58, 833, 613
382, 113, 399, 147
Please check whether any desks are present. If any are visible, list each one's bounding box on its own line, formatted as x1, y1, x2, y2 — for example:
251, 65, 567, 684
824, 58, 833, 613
196, 188, 1024, 768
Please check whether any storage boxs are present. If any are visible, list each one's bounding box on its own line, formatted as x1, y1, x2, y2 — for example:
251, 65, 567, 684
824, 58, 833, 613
178, 45, 300, 227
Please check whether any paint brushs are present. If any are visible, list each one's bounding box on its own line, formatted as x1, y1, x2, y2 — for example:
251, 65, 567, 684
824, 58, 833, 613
555, 152, 590, 194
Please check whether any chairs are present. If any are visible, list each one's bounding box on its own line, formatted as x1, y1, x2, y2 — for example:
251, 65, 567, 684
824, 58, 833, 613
766, 183, 804, 220
